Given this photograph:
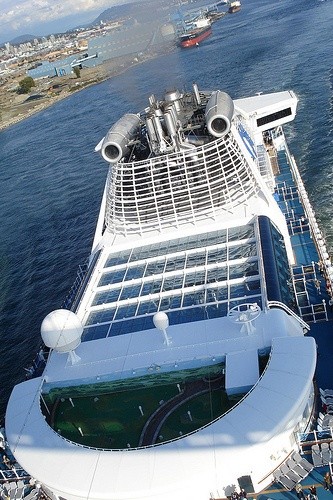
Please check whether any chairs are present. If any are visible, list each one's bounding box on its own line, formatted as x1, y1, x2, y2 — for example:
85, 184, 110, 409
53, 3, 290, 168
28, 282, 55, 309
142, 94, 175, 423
0, 478, 42, 500
273, 388, 333, 491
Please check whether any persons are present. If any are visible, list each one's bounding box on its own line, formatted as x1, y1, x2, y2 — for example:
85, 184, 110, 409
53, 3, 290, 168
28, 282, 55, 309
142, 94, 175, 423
324, 472, 330, 491
0, 447, 14, 470
314, 259, 331, 294
231, 488, 247, 500
40, 493, 45, 500
296, 483, 318, 500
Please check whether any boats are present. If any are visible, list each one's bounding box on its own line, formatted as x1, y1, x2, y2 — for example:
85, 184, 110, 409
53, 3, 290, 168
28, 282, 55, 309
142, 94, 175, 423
1, 83, 333, 500
229, 0, 241, 13
177, 25, 212, 48
176, 6, 226, 32
